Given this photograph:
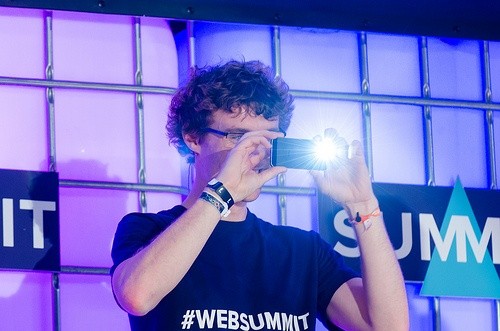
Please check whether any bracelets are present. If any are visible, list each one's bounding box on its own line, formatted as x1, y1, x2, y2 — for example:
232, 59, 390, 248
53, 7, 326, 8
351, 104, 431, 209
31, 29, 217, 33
205, 177, 236, 210
348, 207, 383, 231
198, 191, 232, 219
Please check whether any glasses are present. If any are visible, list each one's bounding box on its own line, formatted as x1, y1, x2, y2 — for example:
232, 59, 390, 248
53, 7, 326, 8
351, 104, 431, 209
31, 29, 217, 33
201, 127, 287, 144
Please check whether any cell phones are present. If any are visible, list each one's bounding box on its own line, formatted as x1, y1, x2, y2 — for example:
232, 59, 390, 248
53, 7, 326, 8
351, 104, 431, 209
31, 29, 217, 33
268, 138, 329, 171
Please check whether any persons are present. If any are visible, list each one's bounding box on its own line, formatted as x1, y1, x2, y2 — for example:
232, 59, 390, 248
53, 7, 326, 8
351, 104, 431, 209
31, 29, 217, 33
110, 60, 411, 331
